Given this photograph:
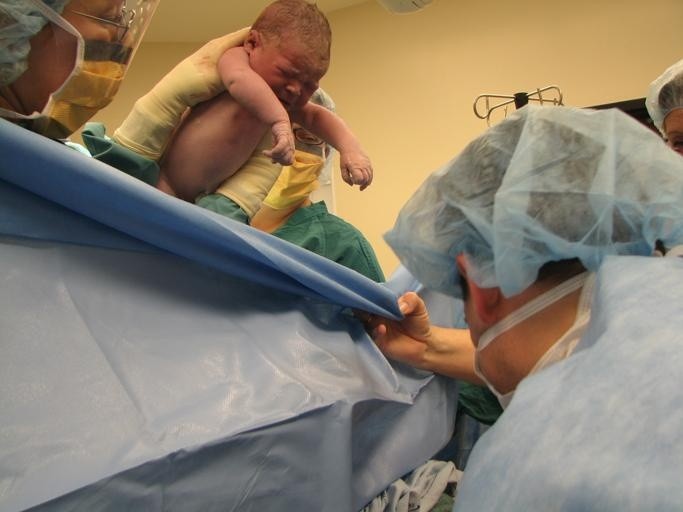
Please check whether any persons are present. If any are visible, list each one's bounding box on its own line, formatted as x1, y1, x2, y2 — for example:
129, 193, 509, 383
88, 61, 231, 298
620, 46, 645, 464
382, 101, 683, 512
360, 58, 683, 387
0, 0, 284, 226
250, 87, 386, 284
156, 0, 374, 202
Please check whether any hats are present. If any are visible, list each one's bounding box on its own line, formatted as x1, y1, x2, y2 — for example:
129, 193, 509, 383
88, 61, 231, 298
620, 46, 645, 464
0, 0, 70, 84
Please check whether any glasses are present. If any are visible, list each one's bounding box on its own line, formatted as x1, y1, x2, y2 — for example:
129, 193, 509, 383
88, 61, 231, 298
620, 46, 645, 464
292, 129, 329, 146
65, 6, 136, 43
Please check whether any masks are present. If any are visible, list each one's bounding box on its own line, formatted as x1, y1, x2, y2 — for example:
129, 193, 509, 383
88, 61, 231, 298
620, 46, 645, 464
470, 268, 589, 410
0, 1, 135, 142
264, 147, 326, 211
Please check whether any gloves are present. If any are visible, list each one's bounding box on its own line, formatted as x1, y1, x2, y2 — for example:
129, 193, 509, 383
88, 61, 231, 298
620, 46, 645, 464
112, 26, 256, 162
213, 132, 283, 219
